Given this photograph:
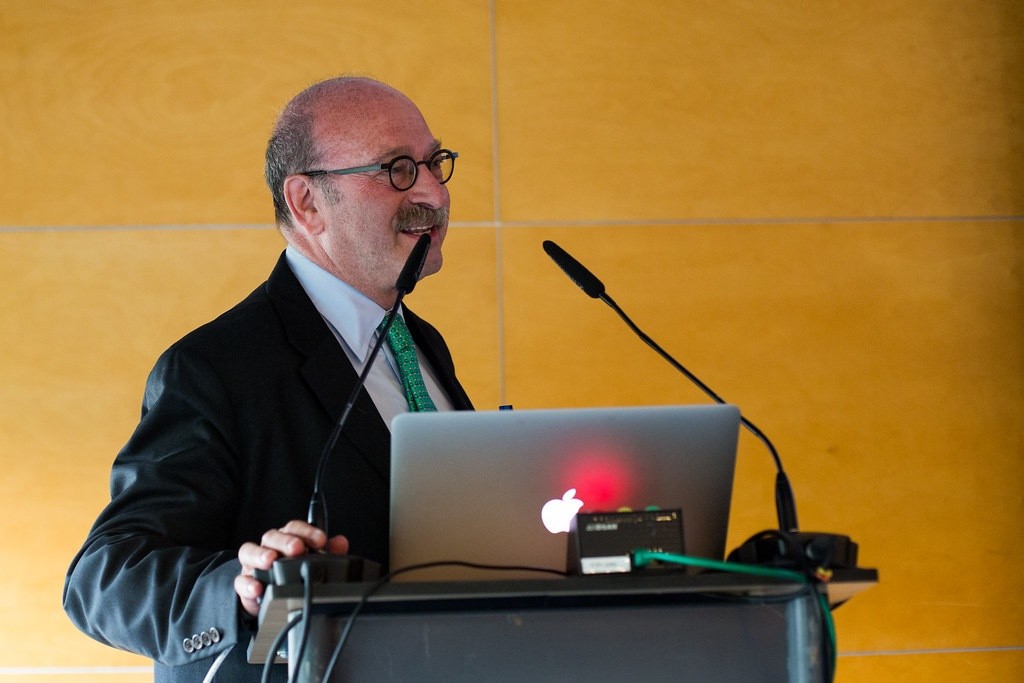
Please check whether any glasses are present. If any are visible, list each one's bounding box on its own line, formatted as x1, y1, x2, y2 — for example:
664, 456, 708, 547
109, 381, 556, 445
295, 148, 459, 192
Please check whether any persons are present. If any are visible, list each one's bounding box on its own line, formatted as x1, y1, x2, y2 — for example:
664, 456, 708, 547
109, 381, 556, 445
61, 76, 476, 683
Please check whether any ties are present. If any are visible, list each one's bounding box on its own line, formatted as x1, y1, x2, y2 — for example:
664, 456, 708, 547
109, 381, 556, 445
376, 312, 438, 414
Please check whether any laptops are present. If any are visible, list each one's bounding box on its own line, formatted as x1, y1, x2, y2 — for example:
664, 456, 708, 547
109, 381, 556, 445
388, 403, 737, 576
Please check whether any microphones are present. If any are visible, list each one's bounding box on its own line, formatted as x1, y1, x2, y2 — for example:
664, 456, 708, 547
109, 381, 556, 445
270, 232, 432, 587
542, 239, 855, 570
